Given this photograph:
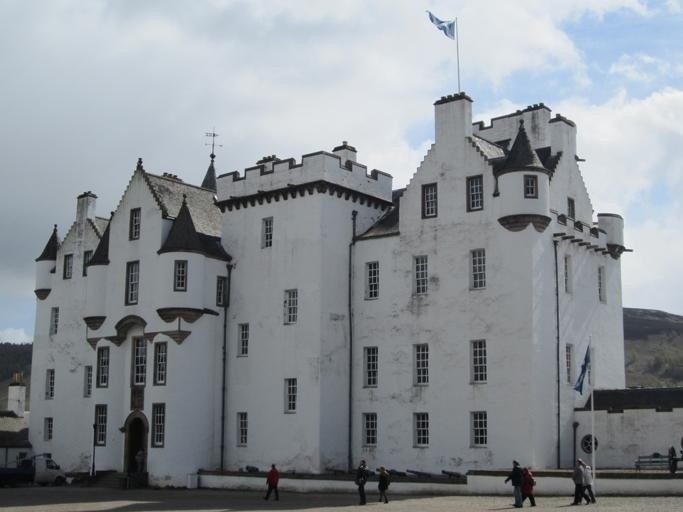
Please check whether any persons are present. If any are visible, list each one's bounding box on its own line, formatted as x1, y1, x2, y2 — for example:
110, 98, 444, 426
135, 447, 144, 472
354, 460, 368, 506
505, 460, 524, 508
511, 467, 536, 507
570, 458, 596, 505
377, 466, 391, 504
262, 464, 279, 501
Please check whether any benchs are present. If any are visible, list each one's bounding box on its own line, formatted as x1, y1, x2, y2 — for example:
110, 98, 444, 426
634, 455, 676, 473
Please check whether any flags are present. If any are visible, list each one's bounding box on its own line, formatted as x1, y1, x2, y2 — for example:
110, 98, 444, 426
426, 10, 455, 41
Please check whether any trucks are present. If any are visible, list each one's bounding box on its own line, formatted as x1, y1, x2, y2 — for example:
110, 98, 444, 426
1, 440, 67, 486
